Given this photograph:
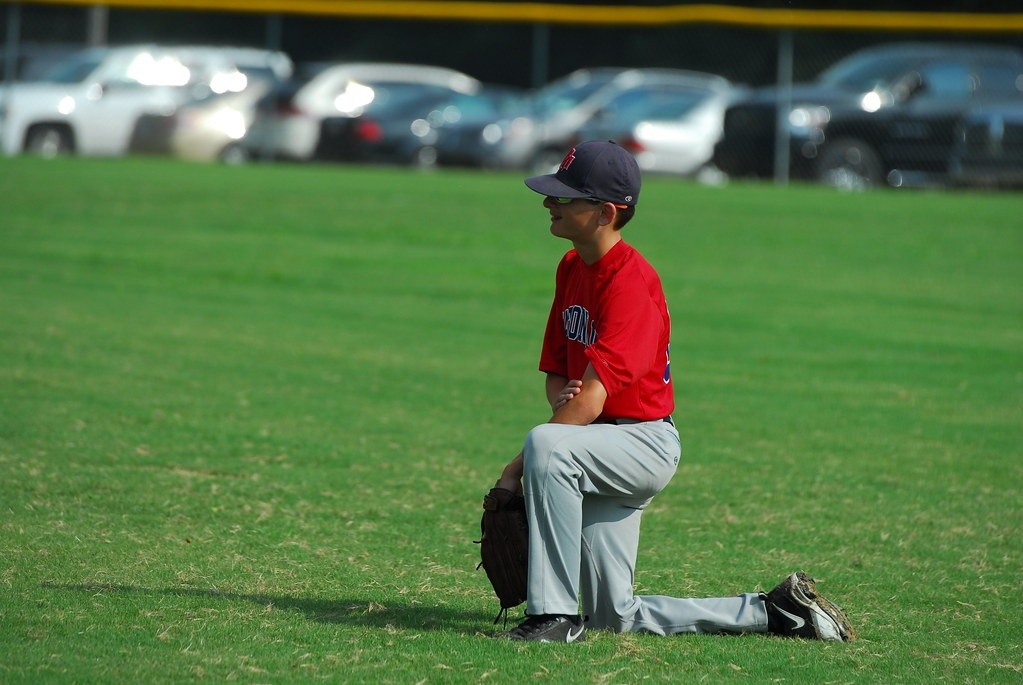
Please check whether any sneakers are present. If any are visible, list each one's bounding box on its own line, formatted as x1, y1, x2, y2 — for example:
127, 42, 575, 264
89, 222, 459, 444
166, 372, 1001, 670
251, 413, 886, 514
757, 569, 855, 642
496, 614, 587, 643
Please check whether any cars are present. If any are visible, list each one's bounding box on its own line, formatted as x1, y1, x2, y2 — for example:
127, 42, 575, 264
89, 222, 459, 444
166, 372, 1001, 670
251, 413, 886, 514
1, 45, 296, 181
315, 62, 754, 189
711, 38, 1023, 196
162, 52, 500, 172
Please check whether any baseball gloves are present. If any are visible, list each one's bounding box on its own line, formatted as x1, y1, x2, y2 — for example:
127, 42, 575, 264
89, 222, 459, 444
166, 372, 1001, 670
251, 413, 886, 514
471, 477, 529, 630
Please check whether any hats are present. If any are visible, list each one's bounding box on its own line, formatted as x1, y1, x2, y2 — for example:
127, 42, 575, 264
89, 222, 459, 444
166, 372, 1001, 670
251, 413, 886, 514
524, 139, 641, 206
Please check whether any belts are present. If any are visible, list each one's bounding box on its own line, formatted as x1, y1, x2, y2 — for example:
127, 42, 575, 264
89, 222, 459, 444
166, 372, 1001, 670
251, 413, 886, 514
603, 413, 674, 426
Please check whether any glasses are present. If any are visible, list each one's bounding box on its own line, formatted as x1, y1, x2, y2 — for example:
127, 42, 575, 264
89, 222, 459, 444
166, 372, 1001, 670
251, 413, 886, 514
554, 196, 628, 208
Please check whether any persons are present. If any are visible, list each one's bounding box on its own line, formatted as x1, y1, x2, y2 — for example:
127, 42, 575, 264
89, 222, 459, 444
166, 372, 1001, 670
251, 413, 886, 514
480, 140, 854, 642
